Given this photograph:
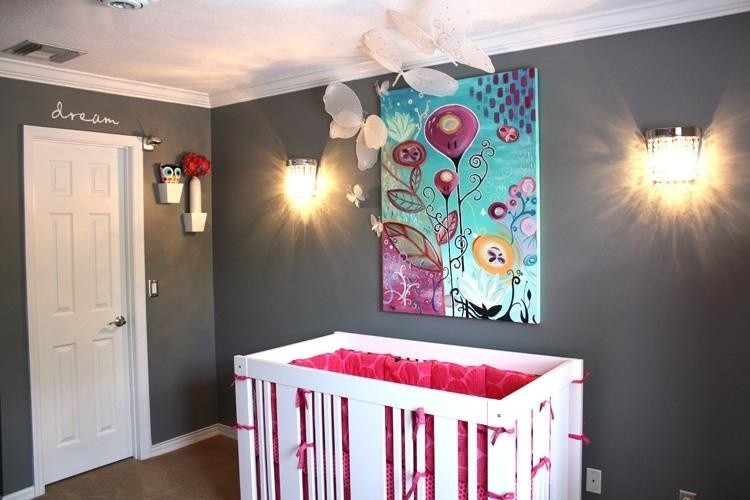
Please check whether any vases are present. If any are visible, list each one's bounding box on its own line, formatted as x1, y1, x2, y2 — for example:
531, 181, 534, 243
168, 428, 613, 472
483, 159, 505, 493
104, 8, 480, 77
189, 176, 202, 213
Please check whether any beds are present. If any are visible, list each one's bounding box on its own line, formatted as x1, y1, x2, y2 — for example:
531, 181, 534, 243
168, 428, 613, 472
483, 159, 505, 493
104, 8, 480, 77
234, 330, 585, 500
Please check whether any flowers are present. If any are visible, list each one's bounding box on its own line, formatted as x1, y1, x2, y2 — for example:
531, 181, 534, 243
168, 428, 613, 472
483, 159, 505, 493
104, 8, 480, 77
179, 152, 212, 177
